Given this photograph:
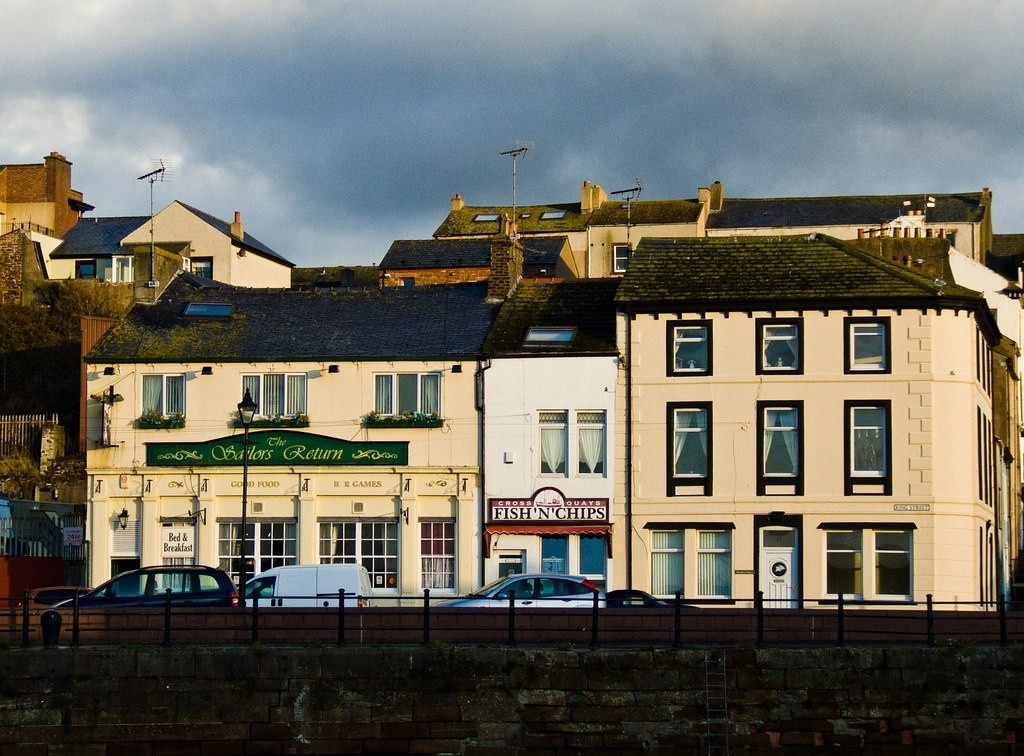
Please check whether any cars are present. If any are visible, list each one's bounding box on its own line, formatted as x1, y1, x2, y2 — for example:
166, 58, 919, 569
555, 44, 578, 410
606, 589, 701, 608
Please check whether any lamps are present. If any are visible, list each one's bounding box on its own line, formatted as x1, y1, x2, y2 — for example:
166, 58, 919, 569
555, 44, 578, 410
118, 508, 129, 530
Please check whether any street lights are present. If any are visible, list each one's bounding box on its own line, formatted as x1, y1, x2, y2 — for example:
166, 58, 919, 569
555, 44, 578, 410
236, 387, 259, 607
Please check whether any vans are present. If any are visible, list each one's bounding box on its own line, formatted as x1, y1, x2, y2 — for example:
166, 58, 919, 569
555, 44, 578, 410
237, 564, 375, 608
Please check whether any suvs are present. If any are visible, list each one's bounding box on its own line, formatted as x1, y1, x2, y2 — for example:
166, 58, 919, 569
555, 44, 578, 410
49, 564, 240, 608
21, 587, 104, 613
430, 573, 608, 609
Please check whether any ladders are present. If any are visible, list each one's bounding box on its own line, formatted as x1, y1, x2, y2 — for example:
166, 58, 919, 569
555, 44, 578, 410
702, 648, 730, 756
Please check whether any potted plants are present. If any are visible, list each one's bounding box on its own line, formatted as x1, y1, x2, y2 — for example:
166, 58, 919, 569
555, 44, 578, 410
134, 408, 186, 429
233, 411, 310, 428
361, 410, 443, 428
